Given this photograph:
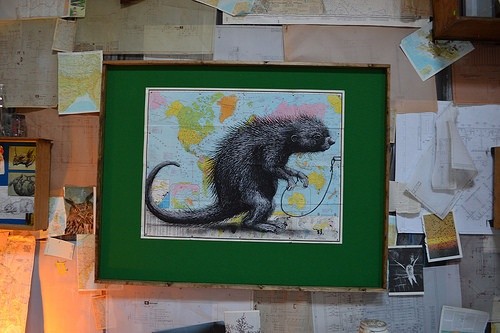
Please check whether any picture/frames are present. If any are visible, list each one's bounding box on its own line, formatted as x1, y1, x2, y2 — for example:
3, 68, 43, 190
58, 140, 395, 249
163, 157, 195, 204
94, 59, 390, 294
